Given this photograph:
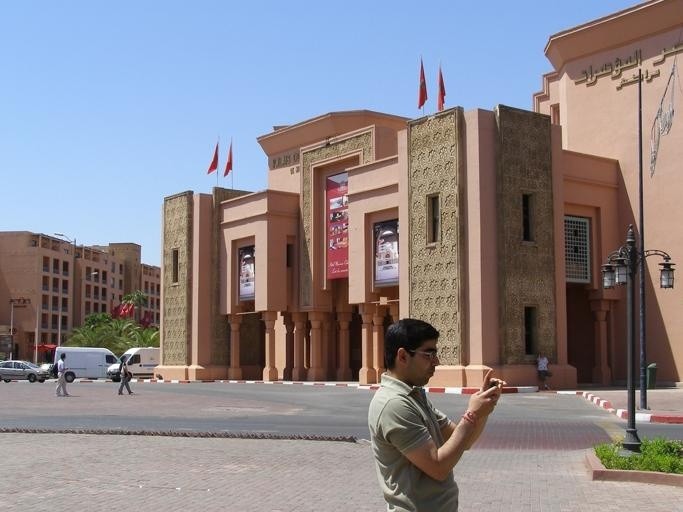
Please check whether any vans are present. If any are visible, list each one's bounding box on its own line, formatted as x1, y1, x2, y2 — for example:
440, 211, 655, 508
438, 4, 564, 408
53, 347, 160, 383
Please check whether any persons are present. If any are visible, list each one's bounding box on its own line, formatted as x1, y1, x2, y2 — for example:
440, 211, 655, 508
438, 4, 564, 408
115, 354, 134, 396
329, 226, 343, 251
535, 347, 553, 391
53, 352, 71, 398
367, 317, 506, 511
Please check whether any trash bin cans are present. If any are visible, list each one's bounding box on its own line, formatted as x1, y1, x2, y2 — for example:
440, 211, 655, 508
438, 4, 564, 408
646, 363, 658, 389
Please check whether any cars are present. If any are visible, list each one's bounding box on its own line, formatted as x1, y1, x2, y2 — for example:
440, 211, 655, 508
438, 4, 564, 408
0, 360, 54, 382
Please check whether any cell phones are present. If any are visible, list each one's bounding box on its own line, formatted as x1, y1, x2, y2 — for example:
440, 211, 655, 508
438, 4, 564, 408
488, 380, 497, 396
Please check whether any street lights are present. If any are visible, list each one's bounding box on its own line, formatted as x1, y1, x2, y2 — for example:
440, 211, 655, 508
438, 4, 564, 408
600, 224, 676, 458
54, 232, 80, 328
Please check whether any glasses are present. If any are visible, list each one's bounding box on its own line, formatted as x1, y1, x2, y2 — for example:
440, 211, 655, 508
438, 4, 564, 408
408, 348, 438, 360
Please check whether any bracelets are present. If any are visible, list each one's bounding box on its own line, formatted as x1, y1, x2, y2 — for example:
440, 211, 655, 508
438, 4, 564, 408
461, 409, 481, 426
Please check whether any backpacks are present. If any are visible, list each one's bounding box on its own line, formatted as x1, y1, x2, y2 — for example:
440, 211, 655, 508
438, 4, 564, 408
50, 363, 58, 379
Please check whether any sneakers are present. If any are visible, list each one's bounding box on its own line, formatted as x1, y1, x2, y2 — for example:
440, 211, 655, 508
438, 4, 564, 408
55, 393, 73, 398
117, 392, 125, 395
128, 392, 134, 395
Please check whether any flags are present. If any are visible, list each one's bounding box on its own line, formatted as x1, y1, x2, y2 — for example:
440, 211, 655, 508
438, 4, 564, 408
205, 141, 218, 175
222, 136, 234, 178
416, 55, 428, 110
436, 59, 446, 114
